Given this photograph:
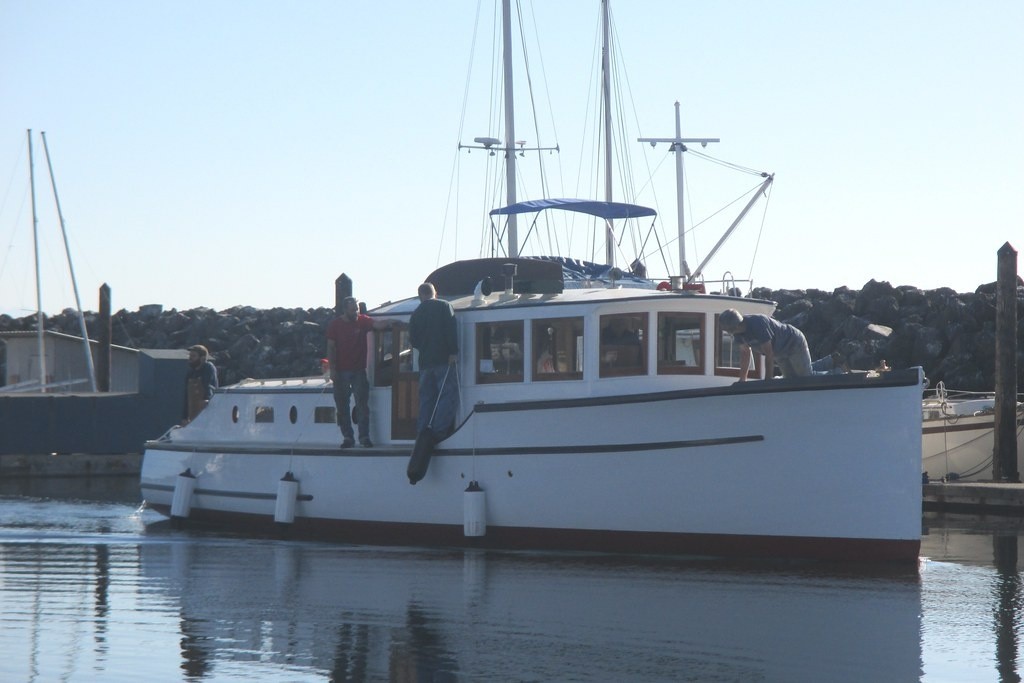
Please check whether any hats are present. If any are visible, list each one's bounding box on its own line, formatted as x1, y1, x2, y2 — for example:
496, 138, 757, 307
187, 345, 207, 356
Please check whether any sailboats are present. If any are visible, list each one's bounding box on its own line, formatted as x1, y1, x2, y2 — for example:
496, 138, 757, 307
138, 1, 929, 570
0, 129, 143, 456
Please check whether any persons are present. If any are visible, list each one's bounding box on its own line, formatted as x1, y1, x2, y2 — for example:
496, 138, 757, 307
180, 345, 218, 427
719, 308, 843, 382
408, 282, 460, 442
600, 312, 643, 368
326, 297, 403, 448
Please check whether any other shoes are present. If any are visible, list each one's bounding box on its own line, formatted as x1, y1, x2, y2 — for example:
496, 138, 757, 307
360, 439, 373, 448
832, 352, 847, 372
341, 438, 355, 448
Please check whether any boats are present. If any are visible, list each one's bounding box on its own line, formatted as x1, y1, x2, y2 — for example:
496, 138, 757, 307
916, 384, 1024, 484
